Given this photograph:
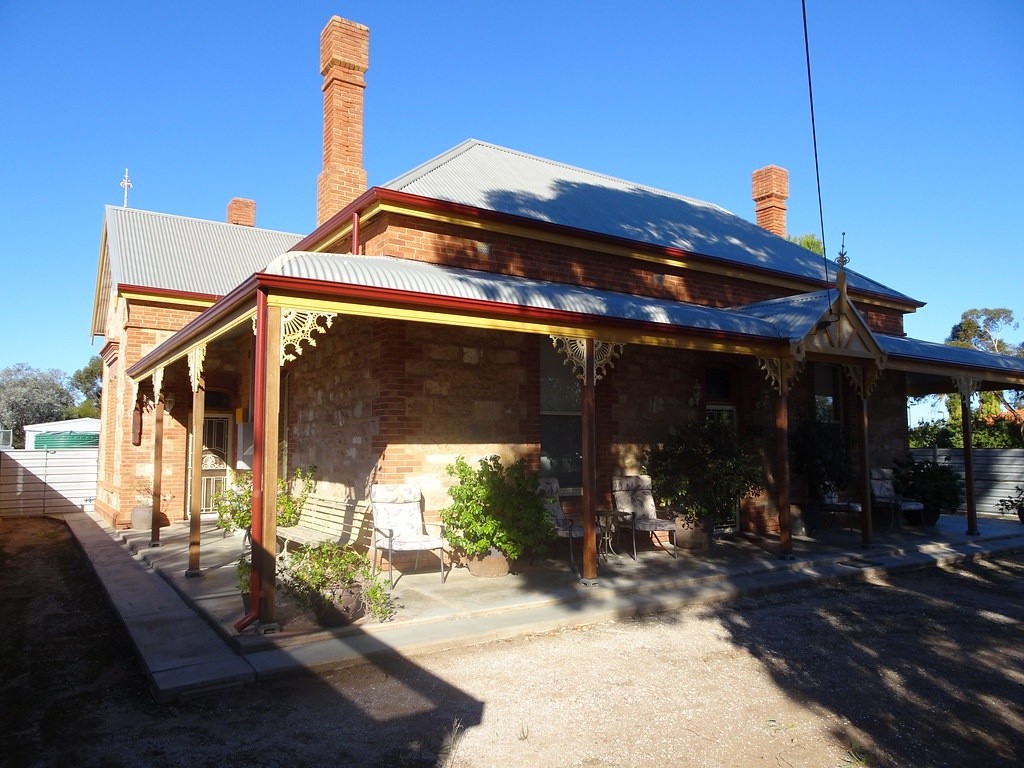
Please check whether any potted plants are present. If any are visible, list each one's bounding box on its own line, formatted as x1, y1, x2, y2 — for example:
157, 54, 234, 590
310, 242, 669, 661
234, 554, 251, 615
778, 399, 859, 536
212, 458, 317, 553
889, 452, 965, 527
642, 414, 765, 550
441, 456, 558, 578
996, 487, 1024, 523
276, 540, 394, 625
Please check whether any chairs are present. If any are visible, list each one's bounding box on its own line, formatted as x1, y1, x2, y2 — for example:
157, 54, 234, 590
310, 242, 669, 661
368, 483, 446, 590
810, 470, 862, 535
612, 475, 678, 560
870, 468, 927, 532
524, 476, 606, 579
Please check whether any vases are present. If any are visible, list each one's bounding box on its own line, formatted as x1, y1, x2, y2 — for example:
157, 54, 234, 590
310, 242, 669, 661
131, 506, 153, 530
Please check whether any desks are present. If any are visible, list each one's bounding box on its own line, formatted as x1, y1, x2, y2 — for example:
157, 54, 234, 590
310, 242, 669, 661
874, 497, 916, 533
584, 510, 631, 556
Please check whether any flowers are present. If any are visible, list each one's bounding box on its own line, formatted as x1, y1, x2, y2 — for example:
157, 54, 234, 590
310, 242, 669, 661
134, 481, 174, 507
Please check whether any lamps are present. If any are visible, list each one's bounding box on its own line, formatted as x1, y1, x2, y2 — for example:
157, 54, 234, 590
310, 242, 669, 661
164, 393, 176, 418
688, 381, 704, 406
